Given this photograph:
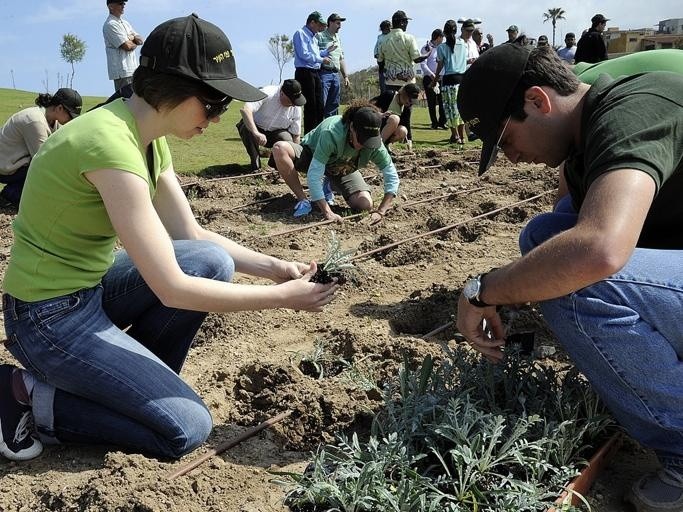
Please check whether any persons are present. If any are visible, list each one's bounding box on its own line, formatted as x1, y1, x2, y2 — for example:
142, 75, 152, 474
556, 32, 578, 67
235, 79, 307, 170
272, 103, 400, 226
103, 0, 143, 96
373, 18, 392, 94
293, 11, 339, 133
311, 14, 350, 121
0, 13, 342, 462
535, 35, 550, 50
367, 82, 421, 155
420, 28, 448, 130
573, 14, 611, 63
3, 88, 82, 207
376, 10, 433, 144
506, 25, 518, 42
473, 28, 491, 56
562, 48, 682, 81
456, 41, 683, 512
432, 18, 469, 144
455, 20, 481, 73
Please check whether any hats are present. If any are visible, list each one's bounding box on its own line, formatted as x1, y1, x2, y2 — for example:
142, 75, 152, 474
53, 88, 83, 119
139, 12, 268, 102
326, 13, 345, 23
106, 0, 128, 6
306, 10, 328, 25
281, 79, 307, 106
591, 14, 610, 24
456, 35, 537, 177
461, 19, 475, 32
392, 10, 412, 21
354, 106, 382, 149
505, 25, 518, 31
538, 35, 548, 43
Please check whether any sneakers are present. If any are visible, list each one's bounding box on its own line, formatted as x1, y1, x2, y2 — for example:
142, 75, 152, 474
292, 198, 313, 218
0, 363, 45, 461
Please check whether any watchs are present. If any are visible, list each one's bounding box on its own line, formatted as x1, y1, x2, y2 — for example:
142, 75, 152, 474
462, 274, 491, 309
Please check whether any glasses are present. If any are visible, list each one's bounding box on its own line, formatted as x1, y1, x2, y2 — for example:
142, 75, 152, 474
117, 2, 126, 6
494, 114, 513, 153
193, 93, 234, 122
